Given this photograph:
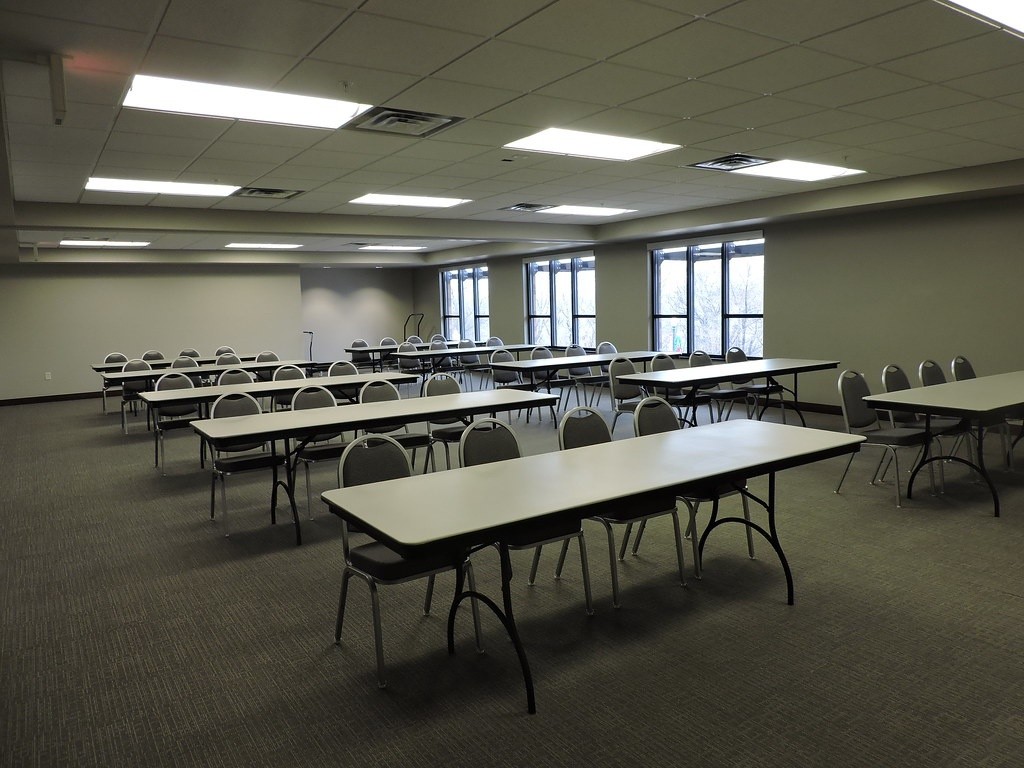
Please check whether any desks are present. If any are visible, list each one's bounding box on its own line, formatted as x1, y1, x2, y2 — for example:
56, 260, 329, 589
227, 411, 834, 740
136, 371, 419, 469
100, 359, 319, 433
861, 370, 1024, 516
488, 351, 663, 430
391, 344, 546, 398
189, 389, 562, 547
321, 417, 867, 715
91, 355, 259, 416
342, 339, 486, 385
615, 357, 840, 430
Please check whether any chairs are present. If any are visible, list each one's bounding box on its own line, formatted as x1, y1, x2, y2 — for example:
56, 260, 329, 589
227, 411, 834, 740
91, 336, 1024, 715
